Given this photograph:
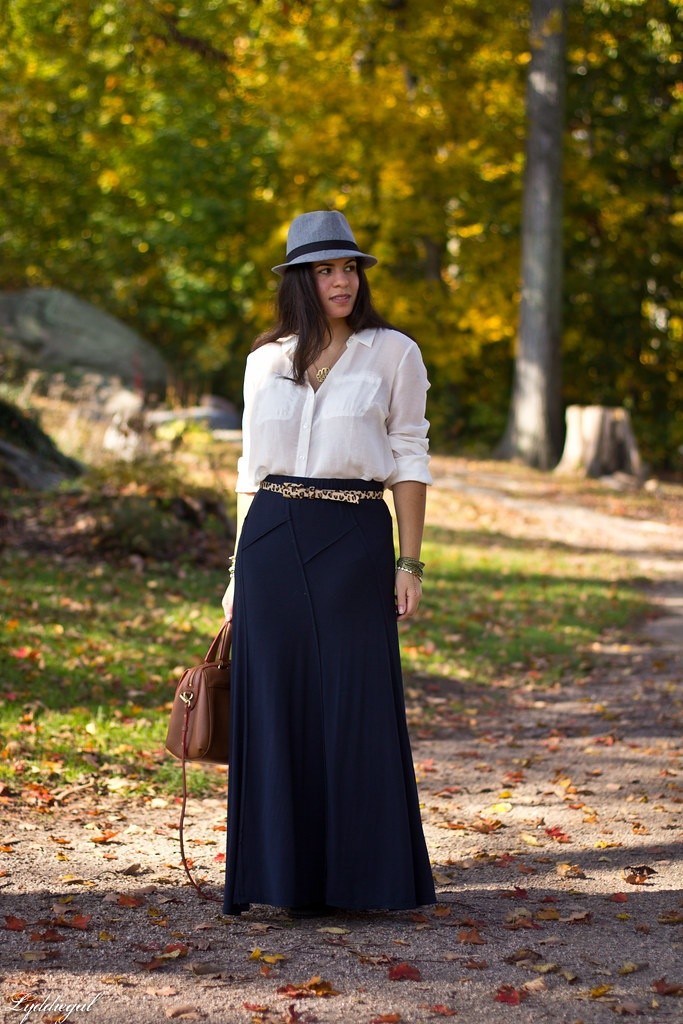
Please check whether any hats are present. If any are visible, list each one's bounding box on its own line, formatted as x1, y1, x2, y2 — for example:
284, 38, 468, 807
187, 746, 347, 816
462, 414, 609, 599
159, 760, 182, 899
270, 210, 378, 276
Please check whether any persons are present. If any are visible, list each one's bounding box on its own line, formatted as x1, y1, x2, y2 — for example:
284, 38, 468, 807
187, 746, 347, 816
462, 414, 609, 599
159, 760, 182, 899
219, 209, 440, 923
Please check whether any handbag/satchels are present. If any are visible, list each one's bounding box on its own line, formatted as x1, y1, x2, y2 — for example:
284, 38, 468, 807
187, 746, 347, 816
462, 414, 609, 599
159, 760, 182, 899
165, 621, 234, 763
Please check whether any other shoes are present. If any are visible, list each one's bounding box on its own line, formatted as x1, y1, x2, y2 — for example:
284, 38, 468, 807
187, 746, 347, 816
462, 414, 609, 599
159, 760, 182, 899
288, 904, 339, 920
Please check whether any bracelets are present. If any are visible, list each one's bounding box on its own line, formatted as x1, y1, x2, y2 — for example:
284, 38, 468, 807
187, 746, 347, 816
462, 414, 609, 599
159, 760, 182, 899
396, 555, 426, 582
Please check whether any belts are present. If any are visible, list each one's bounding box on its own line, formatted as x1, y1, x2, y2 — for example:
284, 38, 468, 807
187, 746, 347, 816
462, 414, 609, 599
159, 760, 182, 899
259, 481, 385, 504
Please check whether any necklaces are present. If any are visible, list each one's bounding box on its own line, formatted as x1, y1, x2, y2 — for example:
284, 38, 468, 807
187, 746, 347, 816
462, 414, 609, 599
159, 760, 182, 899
311, 335, 351, 384
